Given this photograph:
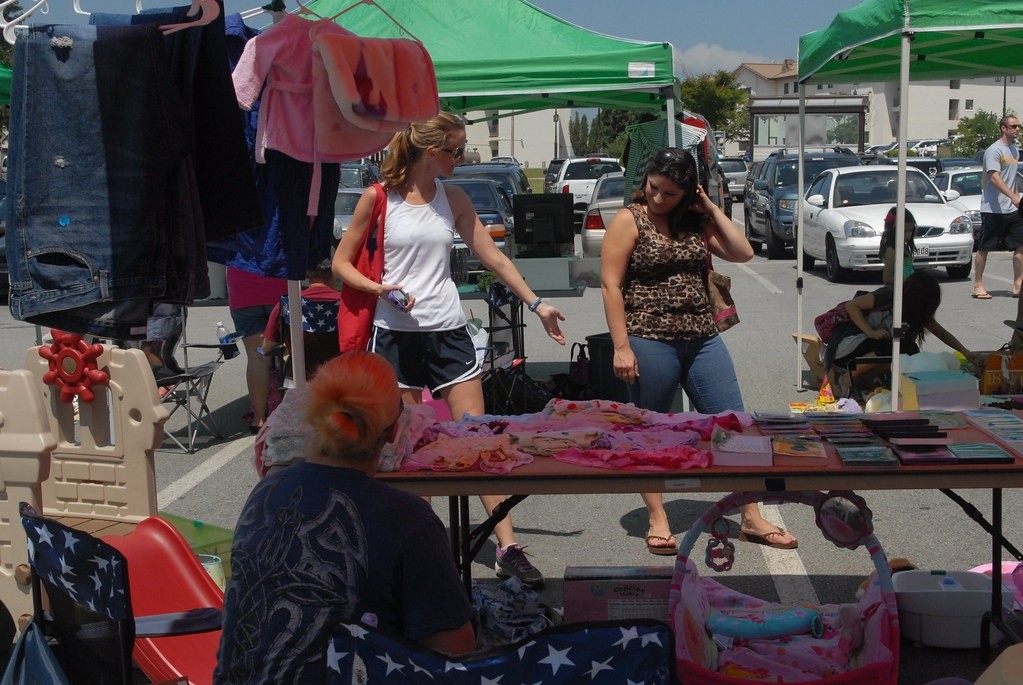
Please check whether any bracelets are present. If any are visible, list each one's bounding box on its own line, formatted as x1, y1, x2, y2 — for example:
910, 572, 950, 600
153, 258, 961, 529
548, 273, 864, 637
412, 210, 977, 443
528, 297, 543, 312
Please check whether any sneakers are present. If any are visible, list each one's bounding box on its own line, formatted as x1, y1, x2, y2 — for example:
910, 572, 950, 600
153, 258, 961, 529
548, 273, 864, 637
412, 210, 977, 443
495, 542, 545, 589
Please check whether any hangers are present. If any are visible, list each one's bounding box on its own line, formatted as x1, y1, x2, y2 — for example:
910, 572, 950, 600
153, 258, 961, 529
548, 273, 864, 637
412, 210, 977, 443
309, 0, 424, 48
625, 101, 708, 144
293, 0, 323, 21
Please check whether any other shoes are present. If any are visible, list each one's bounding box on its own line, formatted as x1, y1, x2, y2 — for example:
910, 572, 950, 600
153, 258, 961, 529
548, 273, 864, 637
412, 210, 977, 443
158, 384, 186, 403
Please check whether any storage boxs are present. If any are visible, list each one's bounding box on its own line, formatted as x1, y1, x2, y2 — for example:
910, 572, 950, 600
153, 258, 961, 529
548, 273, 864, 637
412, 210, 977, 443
977, 351, 1023, 395
562, 564, 676, 625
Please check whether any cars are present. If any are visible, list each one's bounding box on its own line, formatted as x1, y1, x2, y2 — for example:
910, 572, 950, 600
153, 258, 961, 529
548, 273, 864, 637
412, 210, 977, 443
426, 177, 518, 273
790, 164, 977, 280
331, 186, 377, 245
544, 156, 634, 218
854, 140, 988, 181
489, 156, 520, 168
451, 160, 532, 195
340, 163, 386, 188
929, 164, 1023, 240
579, 171, 637, 256
742, 145, 865, 256
714, 156, 750, 196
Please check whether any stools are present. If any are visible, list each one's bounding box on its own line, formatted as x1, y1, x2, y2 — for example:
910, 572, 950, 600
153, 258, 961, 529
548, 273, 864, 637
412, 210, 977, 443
793, 333, 880, 394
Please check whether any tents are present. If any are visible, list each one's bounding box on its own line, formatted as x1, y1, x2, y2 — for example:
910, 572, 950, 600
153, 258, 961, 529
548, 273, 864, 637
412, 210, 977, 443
264, 0, 683, 148
777, 0, 1023, 411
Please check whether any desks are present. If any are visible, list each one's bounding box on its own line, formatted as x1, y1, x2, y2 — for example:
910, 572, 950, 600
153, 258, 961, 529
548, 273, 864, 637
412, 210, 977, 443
372, 407, 1023, 667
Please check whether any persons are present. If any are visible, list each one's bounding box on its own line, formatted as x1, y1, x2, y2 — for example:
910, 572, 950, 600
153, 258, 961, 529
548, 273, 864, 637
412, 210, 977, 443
822, 267, 986, 392
212, 350, 478, 685
972, 115, 1022, 299
227, 249, 339, 433
878, 206, 917, 286
601, 147, 799, 555
331, 110, 566, 591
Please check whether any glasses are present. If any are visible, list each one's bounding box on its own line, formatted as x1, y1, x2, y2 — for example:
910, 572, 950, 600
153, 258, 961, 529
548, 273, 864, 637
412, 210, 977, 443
656, 151, 690, 168
1006, 125, 1021, 128
441, 145, 465, 161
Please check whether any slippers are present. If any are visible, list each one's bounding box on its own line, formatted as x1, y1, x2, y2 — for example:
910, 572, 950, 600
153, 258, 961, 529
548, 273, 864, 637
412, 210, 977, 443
644, 525, 679, 555
829, 383, 859, 399
738, 526, 799, 549
971, 293, 992, 299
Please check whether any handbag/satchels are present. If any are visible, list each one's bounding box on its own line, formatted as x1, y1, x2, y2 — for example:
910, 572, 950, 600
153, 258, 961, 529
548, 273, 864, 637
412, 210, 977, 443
814, 300, 852, 343
338, 183, 388, 355
700, 226, 740, 333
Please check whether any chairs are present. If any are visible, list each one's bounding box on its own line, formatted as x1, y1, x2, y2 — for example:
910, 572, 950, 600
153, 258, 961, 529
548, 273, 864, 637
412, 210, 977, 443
880, 180, 920, 204
2, 503, 224, 685
836, 186, 864, 206
110, 332, 225, 453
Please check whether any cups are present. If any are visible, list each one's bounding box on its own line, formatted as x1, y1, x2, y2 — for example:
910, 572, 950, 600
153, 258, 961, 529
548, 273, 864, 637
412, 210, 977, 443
216, 321, 233, 344
